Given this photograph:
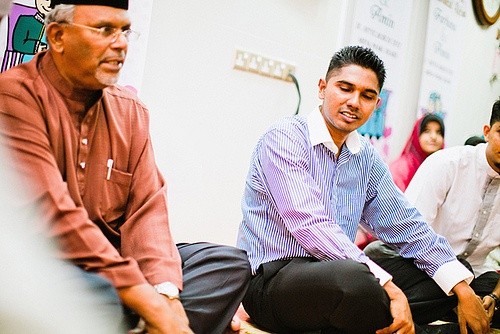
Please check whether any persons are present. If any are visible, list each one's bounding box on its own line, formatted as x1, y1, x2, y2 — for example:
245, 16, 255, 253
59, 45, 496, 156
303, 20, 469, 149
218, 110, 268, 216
363, 96, 500, 329
465, 135, 487, 148
355, 111, 446, 250
237, 47, 491, 334
0, 0, 251, 334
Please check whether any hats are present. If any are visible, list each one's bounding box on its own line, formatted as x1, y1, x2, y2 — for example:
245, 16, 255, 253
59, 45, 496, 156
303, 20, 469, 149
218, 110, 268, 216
48, 0, 129, 9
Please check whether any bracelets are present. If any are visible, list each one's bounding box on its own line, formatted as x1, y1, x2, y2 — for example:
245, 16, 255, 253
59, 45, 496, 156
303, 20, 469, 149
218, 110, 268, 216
489, 292, 500, 304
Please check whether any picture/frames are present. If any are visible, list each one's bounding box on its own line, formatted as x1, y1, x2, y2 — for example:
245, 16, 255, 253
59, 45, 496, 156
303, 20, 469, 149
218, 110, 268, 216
472, 0, 500, 26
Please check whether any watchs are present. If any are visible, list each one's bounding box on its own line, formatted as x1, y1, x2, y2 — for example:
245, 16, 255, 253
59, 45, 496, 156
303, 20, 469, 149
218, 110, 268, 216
153, 282, 182, 302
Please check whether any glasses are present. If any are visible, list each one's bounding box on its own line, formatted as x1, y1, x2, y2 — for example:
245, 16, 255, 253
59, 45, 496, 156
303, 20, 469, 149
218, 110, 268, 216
57, 20, 134, 43
